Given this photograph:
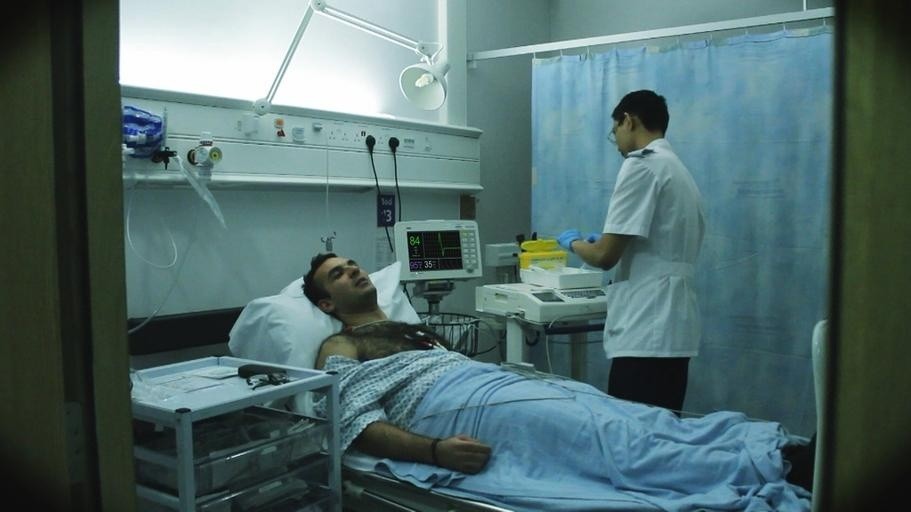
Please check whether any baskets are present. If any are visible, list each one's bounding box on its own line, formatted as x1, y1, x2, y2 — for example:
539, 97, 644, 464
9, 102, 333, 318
417, 312, 479, 357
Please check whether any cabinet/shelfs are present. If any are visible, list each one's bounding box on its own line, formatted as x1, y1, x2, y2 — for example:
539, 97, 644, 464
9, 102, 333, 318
129, 355, 340, 512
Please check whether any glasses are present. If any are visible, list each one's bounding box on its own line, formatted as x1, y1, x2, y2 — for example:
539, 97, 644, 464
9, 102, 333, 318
607, 118, 623, 146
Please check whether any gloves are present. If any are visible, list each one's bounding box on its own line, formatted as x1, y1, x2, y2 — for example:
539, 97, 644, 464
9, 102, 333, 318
557, 229, 601, 251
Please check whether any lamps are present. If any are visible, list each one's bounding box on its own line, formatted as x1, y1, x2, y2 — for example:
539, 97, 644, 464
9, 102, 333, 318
252, 0, 452, 113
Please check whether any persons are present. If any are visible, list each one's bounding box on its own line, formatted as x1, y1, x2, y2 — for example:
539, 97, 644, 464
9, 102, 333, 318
301, 254, 816, 488
558, 91, 709, 415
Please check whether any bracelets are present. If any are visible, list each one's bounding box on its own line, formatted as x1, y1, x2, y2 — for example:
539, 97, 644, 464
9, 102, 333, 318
428, 437, 441, 467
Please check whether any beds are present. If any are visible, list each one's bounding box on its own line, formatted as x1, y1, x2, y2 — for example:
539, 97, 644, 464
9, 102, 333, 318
228, 260, 830, 512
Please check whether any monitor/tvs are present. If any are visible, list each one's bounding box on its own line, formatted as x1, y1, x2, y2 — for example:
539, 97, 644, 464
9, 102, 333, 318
394, 218, 483, 298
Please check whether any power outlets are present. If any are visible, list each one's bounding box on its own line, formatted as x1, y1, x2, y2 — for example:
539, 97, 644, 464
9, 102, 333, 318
329, 124, 400, 153
